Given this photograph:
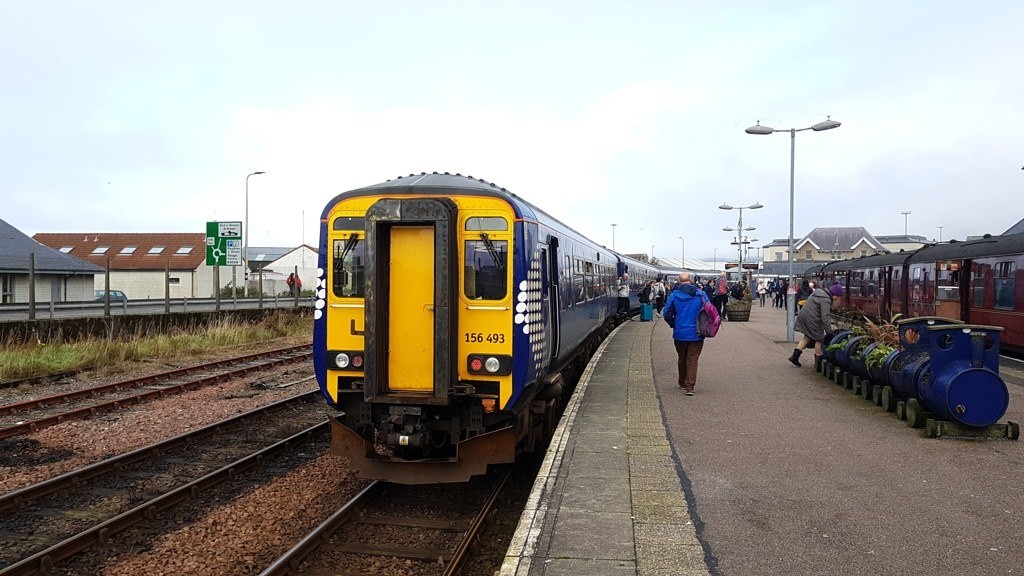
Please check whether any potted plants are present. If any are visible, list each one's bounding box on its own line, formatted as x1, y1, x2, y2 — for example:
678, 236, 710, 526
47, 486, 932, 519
725, 289, 753, 321
815, 302, 1021, 441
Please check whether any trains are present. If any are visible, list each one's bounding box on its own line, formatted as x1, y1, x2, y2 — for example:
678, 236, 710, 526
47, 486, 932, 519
312, 171, 723, 485
796, 232, 1024, 361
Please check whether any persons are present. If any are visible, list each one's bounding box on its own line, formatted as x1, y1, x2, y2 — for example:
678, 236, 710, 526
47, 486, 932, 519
287, 273, 295, 297
788, 284, 844, 372
616, 270, 818, 321
297, 275, 302, 297
662, 273, 711, 395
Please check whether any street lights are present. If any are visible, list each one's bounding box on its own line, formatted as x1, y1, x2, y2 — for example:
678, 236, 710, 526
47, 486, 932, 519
243, 172, 266, 299
744, 115, 842, 345
718, 200, 763, 301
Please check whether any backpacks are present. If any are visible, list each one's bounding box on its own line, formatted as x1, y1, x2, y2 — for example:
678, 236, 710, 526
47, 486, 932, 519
696, 289, 721, 338
287, 277, 294, 285
718, 278, 728, 296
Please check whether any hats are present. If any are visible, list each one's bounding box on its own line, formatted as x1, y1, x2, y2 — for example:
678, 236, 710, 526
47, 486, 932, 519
829, 284, 843, 298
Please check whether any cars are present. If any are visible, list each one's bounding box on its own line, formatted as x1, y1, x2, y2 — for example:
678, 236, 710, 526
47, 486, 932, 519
93, 290, 128, 302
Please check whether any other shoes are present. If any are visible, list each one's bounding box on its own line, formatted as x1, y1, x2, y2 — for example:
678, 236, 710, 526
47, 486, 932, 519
627, 317, 636, 321
656, 313, 660, 318
721, 313, 726, 319
685, 387, 695, 394
680, 385, 686, 389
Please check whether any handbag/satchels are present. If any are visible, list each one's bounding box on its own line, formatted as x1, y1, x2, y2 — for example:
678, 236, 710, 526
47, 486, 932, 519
658, 290, 664, 296
637, 293, 643, 297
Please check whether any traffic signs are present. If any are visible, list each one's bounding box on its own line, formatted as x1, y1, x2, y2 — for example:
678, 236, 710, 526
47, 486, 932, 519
205, 221, 242, 266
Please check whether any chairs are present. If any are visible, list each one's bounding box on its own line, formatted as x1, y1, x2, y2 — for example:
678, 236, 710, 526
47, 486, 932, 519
913, 276, 1016, 309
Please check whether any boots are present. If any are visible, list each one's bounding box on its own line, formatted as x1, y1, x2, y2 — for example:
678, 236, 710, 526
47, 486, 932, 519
788, 349, 802, 367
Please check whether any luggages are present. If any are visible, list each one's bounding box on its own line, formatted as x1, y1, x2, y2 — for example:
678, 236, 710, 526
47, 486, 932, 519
640, 303, 653, 322
